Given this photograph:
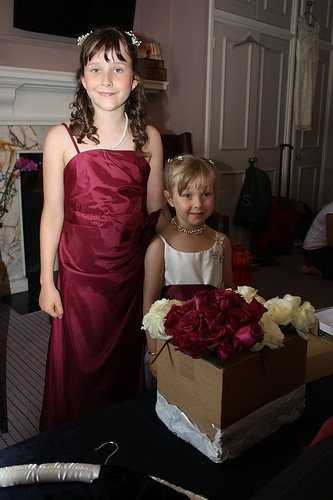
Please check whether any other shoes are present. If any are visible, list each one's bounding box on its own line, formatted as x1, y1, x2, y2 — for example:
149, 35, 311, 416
296, 263, 318, 276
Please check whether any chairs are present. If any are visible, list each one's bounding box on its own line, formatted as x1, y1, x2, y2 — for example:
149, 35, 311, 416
139, 131, 231, 247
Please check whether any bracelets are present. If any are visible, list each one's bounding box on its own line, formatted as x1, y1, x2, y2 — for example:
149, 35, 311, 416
148, 351, 157, 355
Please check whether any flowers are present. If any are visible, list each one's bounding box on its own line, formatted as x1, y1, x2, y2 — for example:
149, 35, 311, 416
138, 283, 321, 362
0, 154, 38, 227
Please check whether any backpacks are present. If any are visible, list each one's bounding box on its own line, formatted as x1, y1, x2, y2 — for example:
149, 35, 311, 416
232, 163, 273, 227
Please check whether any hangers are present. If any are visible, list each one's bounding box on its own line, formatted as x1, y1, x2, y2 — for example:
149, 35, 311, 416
298, 1, 322, 28
231, 28, 276, 54
0, 441, 211, 500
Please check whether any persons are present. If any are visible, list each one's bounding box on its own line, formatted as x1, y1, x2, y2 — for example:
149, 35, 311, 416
37, 25, 172, 436
143, 153, 236, 385
296, 201, 333, 281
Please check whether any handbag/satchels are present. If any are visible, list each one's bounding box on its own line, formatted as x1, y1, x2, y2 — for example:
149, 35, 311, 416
248, 232, 286, 256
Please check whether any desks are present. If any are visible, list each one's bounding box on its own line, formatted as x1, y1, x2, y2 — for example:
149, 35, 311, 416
0, 372, 333, 500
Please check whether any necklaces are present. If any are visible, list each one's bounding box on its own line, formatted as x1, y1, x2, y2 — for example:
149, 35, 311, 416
171, 216, 206, 235
97, 112, 129, 149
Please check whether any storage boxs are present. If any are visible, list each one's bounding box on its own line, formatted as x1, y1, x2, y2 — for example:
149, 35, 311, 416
134, 58, 166, 69
153, 336, 310, 466
305, 306, 333, 387
133, 69, 167, 82
